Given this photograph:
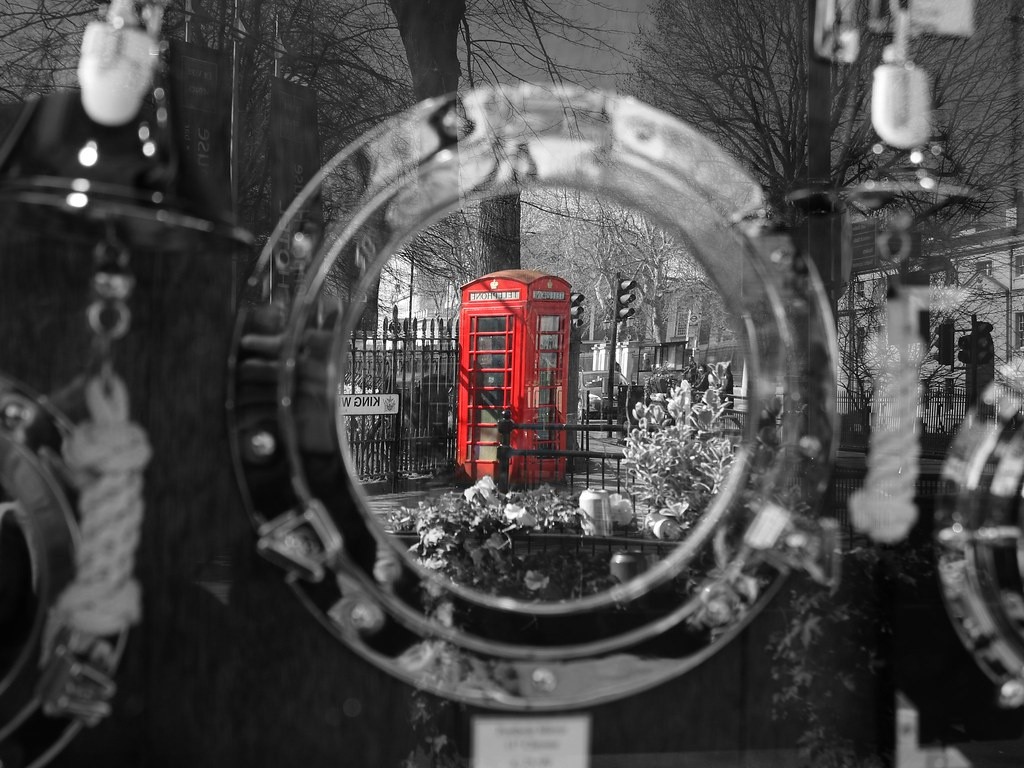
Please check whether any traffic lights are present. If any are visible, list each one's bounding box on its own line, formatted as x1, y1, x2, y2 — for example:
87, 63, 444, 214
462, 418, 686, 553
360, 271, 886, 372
615, 279, 638, 322
569, 293, 585, 331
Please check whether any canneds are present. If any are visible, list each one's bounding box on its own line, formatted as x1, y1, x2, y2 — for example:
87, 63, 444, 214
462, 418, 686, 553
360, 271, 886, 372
610, 551, 647, 587
580, 489, 612, 536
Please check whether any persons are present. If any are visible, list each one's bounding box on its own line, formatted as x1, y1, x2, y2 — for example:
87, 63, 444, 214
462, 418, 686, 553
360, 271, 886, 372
686, 362, 697, 384
695, 362, 709, 393
642, 352, 651, 370
720, 366, 734, 410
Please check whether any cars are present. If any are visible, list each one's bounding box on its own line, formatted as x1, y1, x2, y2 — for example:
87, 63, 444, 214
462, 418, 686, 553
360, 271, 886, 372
578, 371, 636, 419
